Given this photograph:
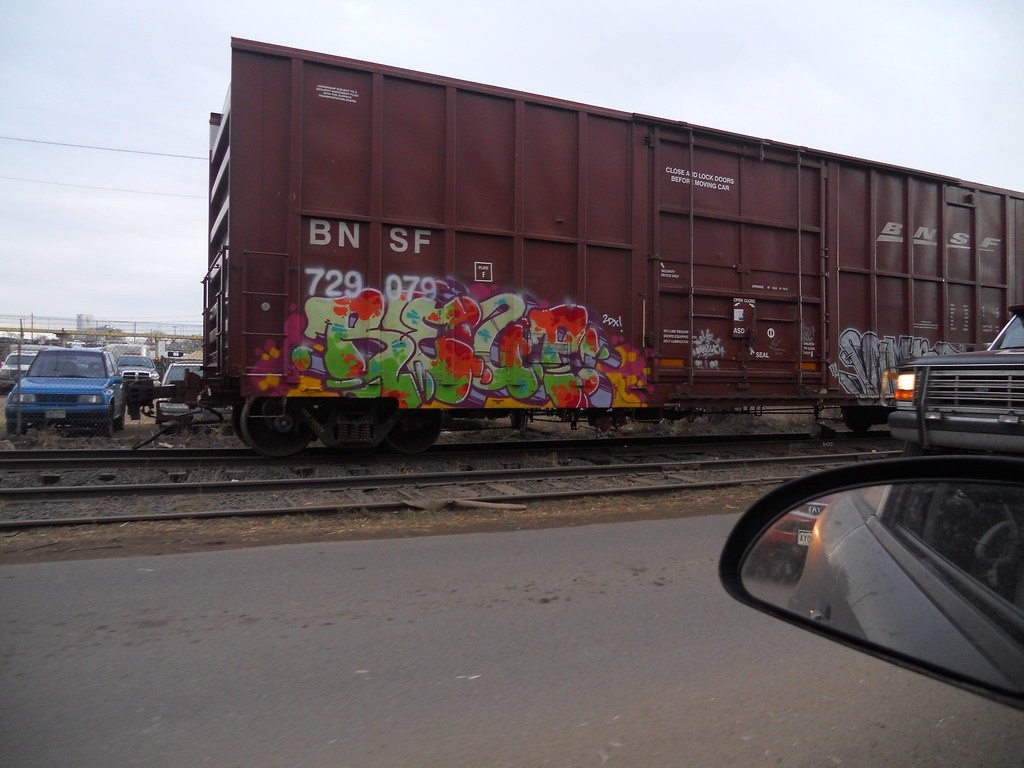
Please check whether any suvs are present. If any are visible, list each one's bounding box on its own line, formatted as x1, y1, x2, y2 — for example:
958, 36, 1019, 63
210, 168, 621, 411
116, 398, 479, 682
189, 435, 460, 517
879, 302, 1024, 456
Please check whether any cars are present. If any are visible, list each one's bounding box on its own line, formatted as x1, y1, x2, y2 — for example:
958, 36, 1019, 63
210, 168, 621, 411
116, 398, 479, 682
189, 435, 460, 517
9, 337, 151, 357
0, 349, 39, 390
154, 363, 205, 426
752, 494, 842, 581
115, 353, 160, 383
3, 346, 125, 438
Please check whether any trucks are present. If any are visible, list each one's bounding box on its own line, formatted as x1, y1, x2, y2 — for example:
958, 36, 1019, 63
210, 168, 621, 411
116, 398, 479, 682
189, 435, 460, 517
196, 35, 1024, 459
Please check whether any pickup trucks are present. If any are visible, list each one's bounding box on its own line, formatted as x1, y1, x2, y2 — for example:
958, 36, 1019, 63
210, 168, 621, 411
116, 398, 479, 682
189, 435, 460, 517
66, 340, 86, 348
